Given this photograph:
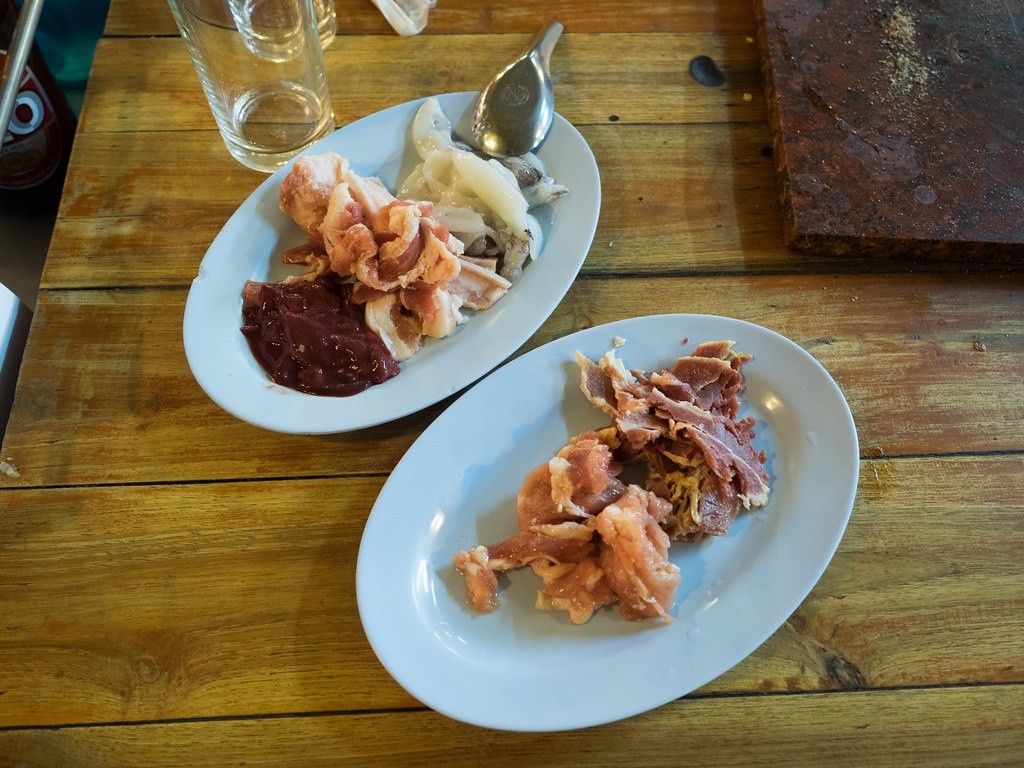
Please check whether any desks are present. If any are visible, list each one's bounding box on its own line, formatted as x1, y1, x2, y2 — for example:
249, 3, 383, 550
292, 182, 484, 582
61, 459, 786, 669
1, 1, 1024, 768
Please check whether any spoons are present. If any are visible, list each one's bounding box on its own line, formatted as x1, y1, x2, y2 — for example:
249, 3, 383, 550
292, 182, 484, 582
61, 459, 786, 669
473, 21, 564, 161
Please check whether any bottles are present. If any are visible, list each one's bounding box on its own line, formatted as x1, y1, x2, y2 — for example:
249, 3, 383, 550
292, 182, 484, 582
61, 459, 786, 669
0, 0, 75, 224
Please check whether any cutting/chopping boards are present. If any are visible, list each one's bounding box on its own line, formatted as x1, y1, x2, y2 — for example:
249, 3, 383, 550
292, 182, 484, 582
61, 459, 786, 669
757, 0, 1024, 260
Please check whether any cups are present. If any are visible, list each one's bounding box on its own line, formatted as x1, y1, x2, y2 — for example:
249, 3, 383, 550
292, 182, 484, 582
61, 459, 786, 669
169, 0, 336, 174
229, 0, 338, 66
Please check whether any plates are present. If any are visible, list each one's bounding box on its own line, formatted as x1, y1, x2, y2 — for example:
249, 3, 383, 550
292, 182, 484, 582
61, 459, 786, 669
354, 314, 860, 733
181, 90, 600, 433
